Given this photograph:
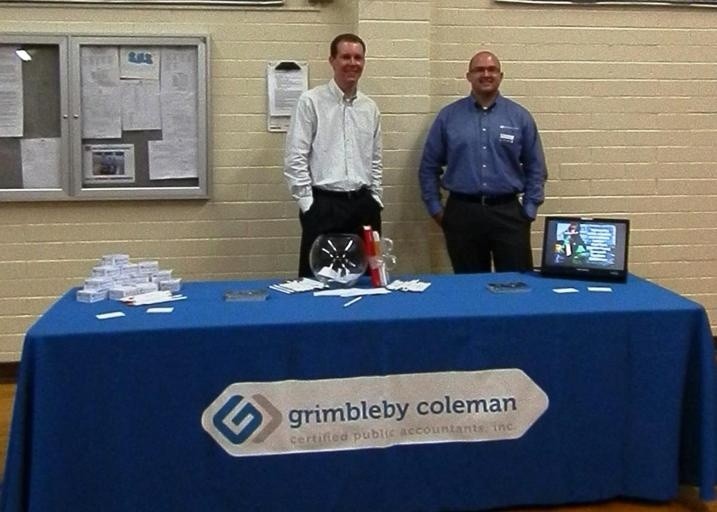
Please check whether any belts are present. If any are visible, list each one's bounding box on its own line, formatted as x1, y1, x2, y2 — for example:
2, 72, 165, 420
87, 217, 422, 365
448, 190, 518, 207
311, 185, 372, 200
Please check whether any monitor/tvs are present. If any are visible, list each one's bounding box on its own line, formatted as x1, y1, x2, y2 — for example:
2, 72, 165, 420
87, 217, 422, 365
541, 215, 631, 284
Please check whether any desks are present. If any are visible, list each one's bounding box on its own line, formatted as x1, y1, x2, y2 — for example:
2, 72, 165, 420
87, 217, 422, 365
6, 270, 717, 512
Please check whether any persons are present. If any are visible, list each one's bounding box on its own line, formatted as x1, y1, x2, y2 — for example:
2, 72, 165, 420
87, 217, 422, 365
417, 50, 547, 272
98, 154, 118, 175
559, 223, 582, 257
283, 33, 384, 280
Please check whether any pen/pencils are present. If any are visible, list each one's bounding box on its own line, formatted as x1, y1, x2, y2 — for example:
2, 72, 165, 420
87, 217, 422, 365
343, 296, 363, 307
268, 283, 295, 294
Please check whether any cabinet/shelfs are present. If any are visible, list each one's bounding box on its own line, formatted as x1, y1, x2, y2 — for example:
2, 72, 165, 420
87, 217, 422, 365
0, 30, 216, 207
